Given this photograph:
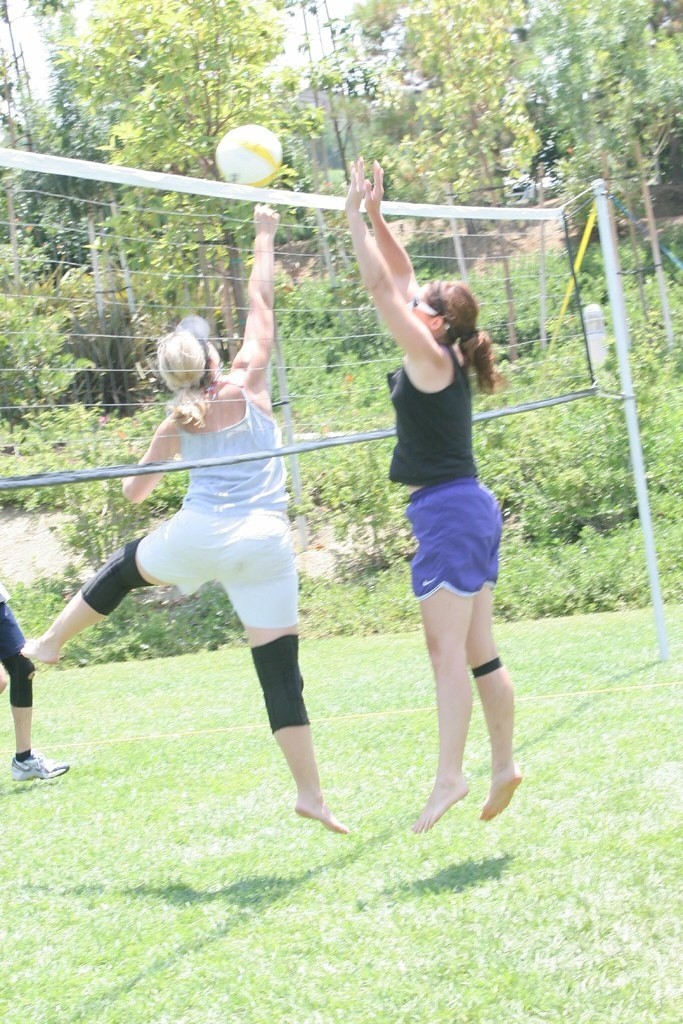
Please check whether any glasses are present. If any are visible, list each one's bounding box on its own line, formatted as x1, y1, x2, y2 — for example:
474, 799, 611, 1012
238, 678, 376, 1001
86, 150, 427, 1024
412, 294, 451, 330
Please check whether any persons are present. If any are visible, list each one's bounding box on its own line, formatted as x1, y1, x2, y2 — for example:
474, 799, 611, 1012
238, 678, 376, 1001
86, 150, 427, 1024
345, 159, 523, 833
22, 206, 349, 833
0, 582, 69, 783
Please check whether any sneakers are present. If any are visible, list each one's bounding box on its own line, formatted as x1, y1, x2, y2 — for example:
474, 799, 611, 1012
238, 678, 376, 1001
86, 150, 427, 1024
11, 750, 70, 781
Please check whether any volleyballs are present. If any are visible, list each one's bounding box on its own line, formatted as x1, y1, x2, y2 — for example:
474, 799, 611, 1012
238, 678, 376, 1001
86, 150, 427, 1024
215, 125, 284, 189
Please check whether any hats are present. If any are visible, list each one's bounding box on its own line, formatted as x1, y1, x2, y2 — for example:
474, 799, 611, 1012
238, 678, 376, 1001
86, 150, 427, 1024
175, 314, 211, 391
